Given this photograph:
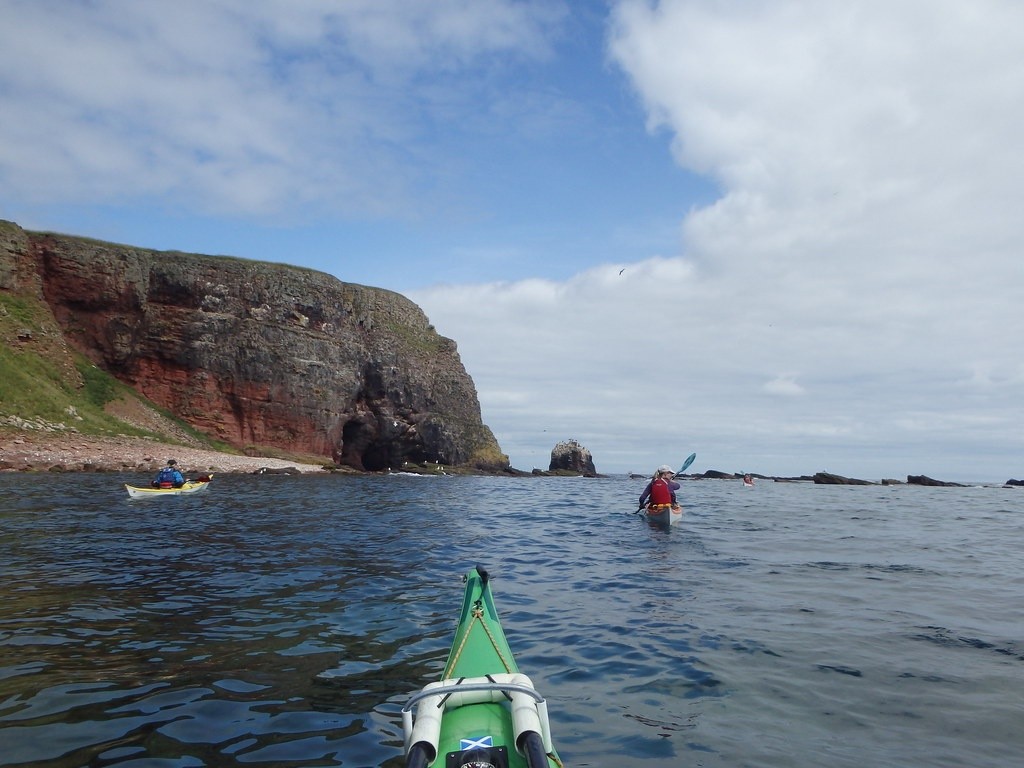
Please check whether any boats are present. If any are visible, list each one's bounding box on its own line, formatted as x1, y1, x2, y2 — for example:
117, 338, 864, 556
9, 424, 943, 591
644, 498, 683, 526
743, 480, 754, 488
125, 473, 214, 498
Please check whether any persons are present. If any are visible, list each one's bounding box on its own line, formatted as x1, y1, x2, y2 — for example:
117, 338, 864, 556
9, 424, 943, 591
156, 460, 186, 488
639, 465, 680, 509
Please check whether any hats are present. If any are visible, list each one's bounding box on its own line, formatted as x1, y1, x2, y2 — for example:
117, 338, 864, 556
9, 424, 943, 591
169, 460, 178, 465
657, 465, 675, 474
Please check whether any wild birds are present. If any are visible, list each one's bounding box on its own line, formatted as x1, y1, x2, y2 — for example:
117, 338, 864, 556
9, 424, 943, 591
618, 268, 626, 276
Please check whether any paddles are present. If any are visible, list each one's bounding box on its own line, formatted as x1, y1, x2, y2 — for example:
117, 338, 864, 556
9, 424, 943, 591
151, 474, 211, 484
634, 453, 696, 515
